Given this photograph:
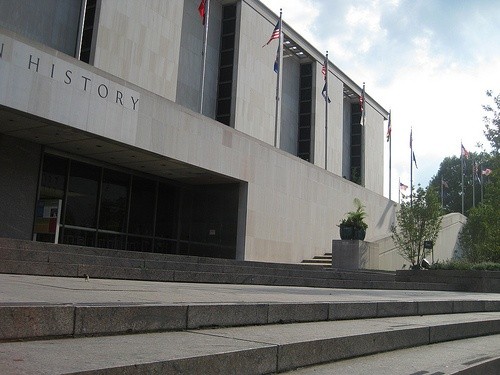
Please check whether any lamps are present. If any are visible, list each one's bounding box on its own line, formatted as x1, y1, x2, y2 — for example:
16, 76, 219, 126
421, 259, 430, 268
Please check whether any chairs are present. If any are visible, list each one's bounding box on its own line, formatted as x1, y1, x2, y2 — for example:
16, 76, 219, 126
64, 235, 113, 249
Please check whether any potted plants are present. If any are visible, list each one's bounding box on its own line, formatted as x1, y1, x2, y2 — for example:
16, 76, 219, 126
337, 197, 368, 240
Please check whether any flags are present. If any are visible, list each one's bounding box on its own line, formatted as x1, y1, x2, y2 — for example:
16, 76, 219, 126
462, 144, 469, 160
442, 176, 450, 188
413, 151, 418, 169
359, 90, 364, 126
320, 57, 331, 104
274, 47, 279, 73
198, 0, 206, 26
387, 112, 391, 142
399, 183, 408, 191
262, 19, 280, 48
